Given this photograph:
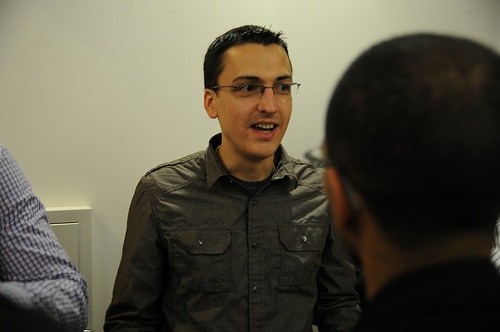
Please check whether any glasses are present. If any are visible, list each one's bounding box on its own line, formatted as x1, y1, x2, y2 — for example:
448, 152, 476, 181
303, 145, 335, 169
211, 83, 301, 99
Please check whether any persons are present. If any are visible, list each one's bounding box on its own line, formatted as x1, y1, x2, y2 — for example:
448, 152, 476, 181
321, 33, 500, 332
98, 24, 363, 332
0, 145, 90, 332
